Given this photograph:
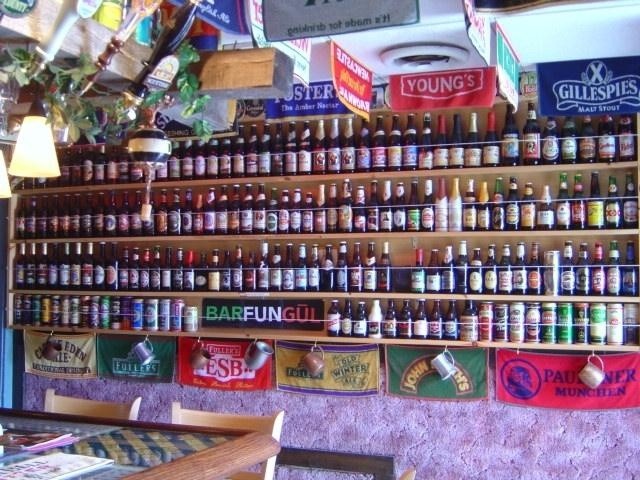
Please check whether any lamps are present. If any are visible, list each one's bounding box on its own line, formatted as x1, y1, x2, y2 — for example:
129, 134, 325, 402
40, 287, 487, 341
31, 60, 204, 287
7, 114, 62, 179
0, 150, 12, 198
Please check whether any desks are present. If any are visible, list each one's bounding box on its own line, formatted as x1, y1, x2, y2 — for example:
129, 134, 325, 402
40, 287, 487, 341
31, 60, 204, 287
1, 406, 283, 480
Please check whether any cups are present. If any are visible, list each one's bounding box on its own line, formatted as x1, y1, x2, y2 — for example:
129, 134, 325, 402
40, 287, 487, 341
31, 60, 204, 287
39, 332, 61, 360
243, 342, 275, 371
578, 355, 606, 392
304, 346, 327, 379
134, 341, 157, 367
189, 342, 211, 371
429, 348, 458, 382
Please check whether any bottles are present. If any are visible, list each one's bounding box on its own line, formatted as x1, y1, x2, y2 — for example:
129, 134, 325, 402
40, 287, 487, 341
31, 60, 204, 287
326, 298, 480, 343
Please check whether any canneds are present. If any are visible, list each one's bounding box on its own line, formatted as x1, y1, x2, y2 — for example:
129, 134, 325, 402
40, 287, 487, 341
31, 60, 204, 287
52, 295, 61, 324
558, 302, 574, 345
624, 303, 640, 346
81, 296, 90, 326
184, 306, 199, 332
70, 295, 81, 325
23, 294, 32, 324
160, 298, 171, 331
494, 304, 510, 342
120, 297, 131, 330
111, 296, 121, 330
61, 296, 70, 324
14, 294, 23, 324
607, 303, 623, 345
99, 296, 111, 330
145, 299, 160, 331
90, 296, 99, 329
478, 302, 494, 342
510, 302, 525, 343
131, 298, 145, 331
590, 303, 607, 346
32, 294, 41, 324
171, 298, 184, 332
41, 295, 52, 324
541, 302, 558, 344
575, 302, 590, 346
525, 302, 542, 344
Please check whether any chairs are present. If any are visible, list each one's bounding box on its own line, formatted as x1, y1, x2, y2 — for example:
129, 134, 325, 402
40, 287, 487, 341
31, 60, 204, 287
42, 387, 142, 422
171, 400, 285, 480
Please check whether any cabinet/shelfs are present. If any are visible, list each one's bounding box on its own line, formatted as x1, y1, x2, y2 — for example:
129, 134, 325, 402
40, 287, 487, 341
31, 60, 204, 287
6, 106, 640, 353
